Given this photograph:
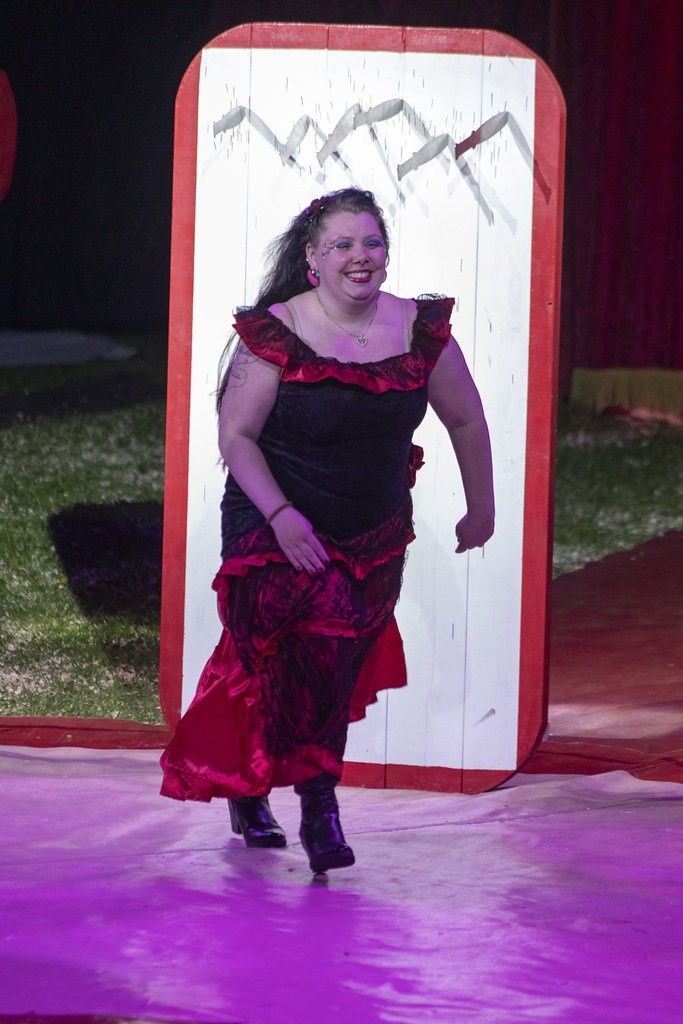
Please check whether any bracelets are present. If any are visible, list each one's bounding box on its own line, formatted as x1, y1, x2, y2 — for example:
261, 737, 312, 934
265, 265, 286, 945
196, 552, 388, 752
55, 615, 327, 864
266, 502, 293, 526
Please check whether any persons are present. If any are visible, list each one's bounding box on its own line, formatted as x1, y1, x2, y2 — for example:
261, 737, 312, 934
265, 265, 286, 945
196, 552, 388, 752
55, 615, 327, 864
158, 188, 495, 873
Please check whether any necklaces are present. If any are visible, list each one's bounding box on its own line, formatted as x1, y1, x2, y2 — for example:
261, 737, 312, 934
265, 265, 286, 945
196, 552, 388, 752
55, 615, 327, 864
315, 288, 378, 346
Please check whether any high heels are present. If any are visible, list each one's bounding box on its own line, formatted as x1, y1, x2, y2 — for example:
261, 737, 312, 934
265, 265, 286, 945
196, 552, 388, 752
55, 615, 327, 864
300, 786, 355, 874
227, 792, 287, 849
